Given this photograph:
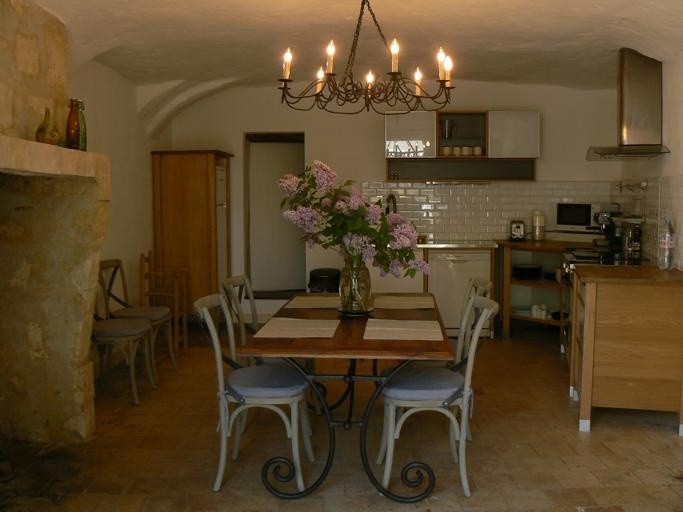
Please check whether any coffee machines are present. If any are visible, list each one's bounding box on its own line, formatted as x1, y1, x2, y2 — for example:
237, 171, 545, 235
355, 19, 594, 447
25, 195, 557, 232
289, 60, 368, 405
586, 212, 623, 250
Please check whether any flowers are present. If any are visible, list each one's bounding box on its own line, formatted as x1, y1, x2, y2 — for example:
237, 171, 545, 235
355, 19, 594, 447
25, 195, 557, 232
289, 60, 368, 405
277, 159, 432, 311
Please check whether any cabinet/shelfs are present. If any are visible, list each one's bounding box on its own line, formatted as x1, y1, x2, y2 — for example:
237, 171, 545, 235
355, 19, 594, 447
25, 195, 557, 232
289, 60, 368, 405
383, 111, 540, 159
153, 148, 234, 325
568, 271, 683, 436
364, 249, 494, 337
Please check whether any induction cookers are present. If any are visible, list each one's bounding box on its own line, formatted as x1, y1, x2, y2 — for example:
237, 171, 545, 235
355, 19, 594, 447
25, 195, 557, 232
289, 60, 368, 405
560, 248, 655, 276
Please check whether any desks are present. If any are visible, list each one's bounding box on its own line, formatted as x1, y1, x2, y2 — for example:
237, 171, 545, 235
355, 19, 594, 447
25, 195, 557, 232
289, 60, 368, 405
503, 240, 611, 354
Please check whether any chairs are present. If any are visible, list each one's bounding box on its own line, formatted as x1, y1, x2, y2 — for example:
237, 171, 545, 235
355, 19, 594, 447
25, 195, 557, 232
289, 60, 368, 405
95, 252, 188, 400
195, 274, 321, 494
382, 281, 497, 496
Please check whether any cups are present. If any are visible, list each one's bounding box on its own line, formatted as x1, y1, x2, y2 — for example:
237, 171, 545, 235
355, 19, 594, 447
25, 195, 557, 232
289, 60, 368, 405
439, 146, 482, 157
440, 118, 456, 139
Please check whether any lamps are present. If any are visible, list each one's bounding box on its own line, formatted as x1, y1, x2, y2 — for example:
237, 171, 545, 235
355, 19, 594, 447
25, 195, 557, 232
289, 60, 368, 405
277, 0, 456, 115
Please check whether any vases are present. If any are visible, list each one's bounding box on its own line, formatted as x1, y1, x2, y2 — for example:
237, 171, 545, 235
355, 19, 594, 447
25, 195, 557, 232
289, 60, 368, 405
340, 254, 369, 317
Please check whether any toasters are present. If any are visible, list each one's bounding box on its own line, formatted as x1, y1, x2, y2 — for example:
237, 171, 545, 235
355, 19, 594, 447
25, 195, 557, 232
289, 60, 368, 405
510, 220, 526, 241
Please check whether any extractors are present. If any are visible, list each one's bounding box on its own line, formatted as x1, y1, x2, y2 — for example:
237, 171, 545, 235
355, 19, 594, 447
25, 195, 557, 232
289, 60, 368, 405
586, 53, 670, 161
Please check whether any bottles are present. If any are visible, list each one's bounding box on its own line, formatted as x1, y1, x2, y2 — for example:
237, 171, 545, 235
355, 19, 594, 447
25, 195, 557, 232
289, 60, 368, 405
633, 225, 642, 253
533, 209, 546, 240
531, 303, 548, 320
658, 219, 677, 270
68, 96, 87, 152
